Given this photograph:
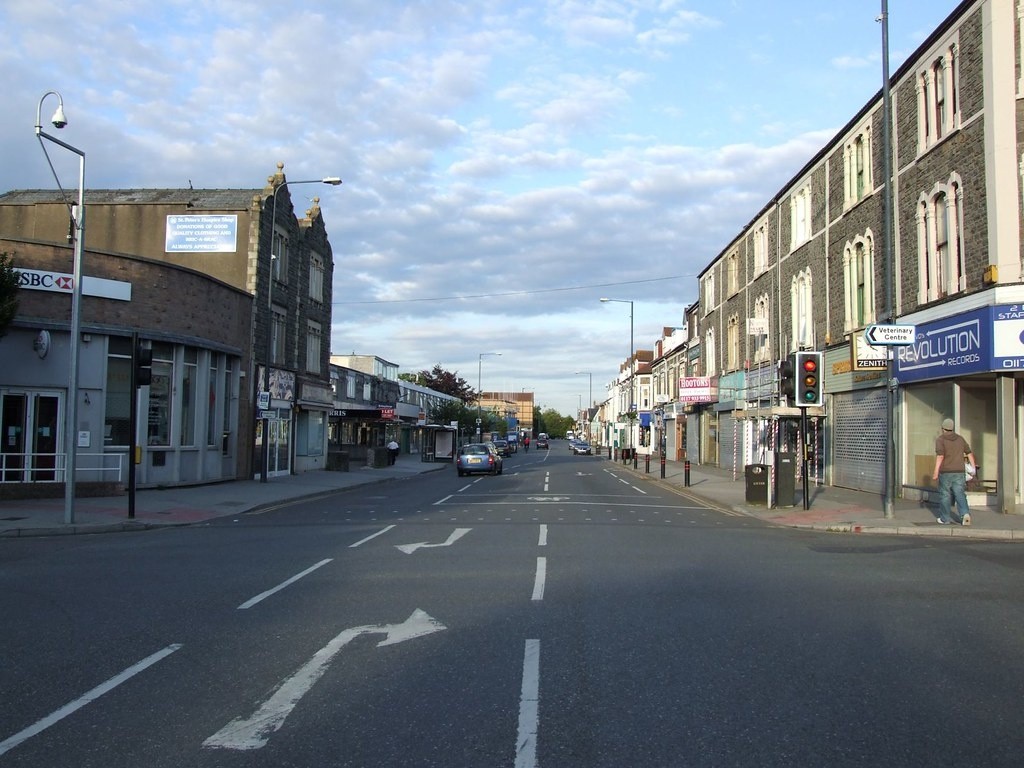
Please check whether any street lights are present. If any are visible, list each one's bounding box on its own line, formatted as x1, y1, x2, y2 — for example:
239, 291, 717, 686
600, 296, 635, 463
476, 353, 503, 443
575, 371, 592, 446
521, 387, 535, 428
260, 177, 343, 483
35, 90, 88, 525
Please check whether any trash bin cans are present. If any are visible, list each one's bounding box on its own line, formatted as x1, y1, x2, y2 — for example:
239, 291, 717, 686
519, 437, 523, 447
622, 448, 636, 459
608, 446, 612, 459
595, 445, 601, 455
327, 451, 349, 472
745, 464, 768, 504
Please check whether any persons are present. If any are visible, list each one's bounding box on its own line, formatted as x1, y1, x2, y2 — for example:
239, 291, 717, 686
933, 419, 975, 526
524, 437, 530, 448
387, 440, 399, 465
523, 433, 527, 439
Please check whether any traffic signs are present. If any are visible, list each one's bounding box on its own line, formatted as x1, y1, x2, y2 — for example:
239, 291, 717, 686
864, 324, 915, 346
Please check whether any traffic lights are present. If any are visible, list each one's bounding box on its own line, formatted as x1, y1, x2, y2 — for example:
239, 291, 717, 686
794, 351, 823, 408
780, 354, 796, 407
130, 332, 155, 386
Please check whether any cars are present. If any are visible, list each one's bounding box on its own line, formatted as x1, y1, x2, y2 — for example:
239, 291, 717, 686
536, 433, 550, 450
482, 439, 518, 458
565, 430, 592, 455
456, 443, 503, 477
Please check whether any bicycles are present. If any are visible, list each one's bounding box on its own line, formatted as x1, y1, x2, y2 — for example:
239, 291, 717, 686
523, 443, 529, 453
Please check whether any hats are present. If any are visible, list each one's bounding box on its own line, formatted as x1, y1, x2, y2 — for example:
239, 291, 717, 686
942, 419, 954, 430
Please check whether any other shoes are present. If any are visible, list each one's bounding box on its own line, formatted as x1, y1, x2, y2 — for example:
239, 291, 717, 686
962, 514, 970, 526
937, 518, 950, 525
